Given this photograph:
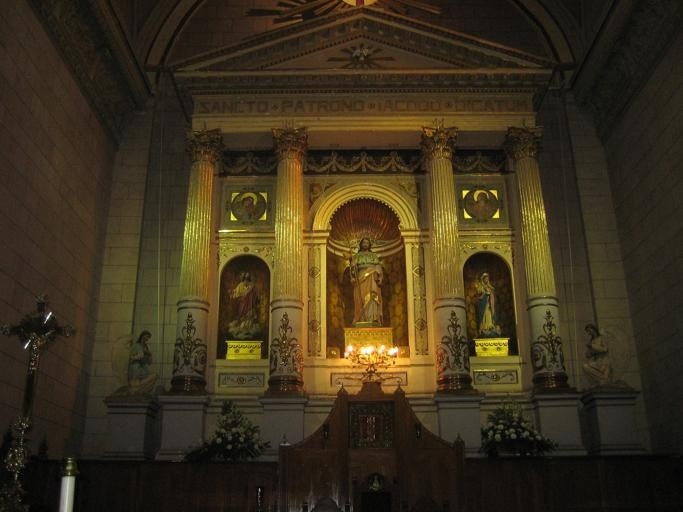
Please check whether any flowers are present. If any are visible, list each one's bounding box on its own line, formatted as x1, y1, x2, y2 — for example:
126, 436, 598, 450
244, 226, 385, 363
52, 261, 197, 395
478, 392, 558, 460
207, 399, 270, 462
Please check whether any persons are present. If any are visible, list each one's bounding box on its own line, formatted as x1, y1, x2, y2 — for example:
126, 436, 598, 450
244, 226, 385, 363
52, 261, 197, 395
476, 271, 501, 337
228, 272, 260, 341
582, 324, 613, 386
349, 238, 385, 326
128, 330, 158, 393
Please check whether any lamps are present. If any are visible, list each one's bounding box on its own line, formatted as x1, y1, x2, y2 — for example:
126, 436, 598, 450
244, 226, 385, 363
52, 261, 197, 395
344, 343, 400, 382
